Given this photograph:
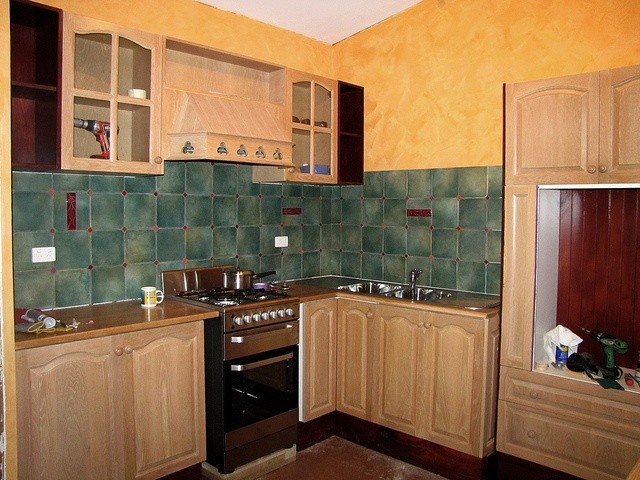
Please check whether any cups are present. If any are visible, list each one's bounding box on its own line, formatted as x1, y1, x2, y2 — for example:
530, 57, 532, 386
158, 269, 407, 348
127, 89, 146, 100
143, 307, 165, 322
139, 286, 164, 308
634, 368, 640, 382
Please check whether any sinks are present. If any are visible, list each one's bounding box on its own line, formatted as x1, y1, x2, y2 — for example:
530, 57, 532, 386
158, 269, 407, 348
384, 288, 453, 301
338, 281, 403, 294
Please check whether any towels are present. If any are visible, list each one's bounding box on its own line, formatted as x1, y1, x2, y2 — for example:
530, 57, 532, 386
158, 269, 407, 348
543, 324, 584, 369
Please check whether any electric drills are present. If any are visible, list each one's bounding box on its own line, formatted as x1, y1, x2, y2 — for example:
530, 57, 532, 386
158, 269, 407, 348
74, 118, 119, 159
581, 327, 629, 380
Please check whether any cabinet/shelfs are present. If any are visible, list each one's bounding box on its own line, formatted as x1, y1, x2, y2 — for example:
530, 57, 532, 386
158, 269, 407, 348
301, 298, 337, 423
10, 0, 63, 173
337, 298, 369, 421
503, 64, 640, 185
370, 298, 501, 459
61, 9, 164, 176
15, 295, 219, 480
339, 81, 365, 185
495, 365, 640, 480
286, 68, 338, 185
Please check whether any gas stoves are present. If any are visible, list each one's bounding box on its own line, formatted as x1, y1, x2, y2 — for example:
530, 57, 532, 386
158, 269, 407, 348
161, 265, 301, 332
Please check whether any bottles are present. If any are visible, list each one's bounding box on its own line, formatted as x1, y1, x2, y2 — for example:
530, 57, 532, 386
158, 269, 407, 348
555, 339, 569, 369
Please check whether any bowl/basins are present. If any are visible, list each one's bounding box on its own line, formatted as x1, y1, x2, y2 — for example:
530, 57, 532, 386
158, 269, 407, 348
253, 282, 268, 291
299, 164, 328, 175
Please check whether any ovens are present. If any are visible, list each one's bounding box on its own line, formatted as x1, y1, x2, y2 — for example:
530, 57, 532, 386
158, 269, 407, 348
224, 320, 300, 452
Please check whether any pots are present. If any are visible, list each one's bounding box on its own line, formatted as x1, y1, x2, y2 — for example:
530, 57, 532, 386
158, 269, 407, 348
223, 266, 276, 289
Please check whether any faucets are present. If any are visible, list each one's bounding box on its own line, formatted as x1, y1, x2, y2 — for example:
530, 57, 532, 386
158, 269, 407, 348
410, 268, 423, 288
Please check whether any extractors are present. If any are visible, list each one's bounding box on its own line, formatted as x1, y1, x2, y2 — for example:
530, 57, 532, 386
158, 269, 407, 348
165, 92, 295, 167
